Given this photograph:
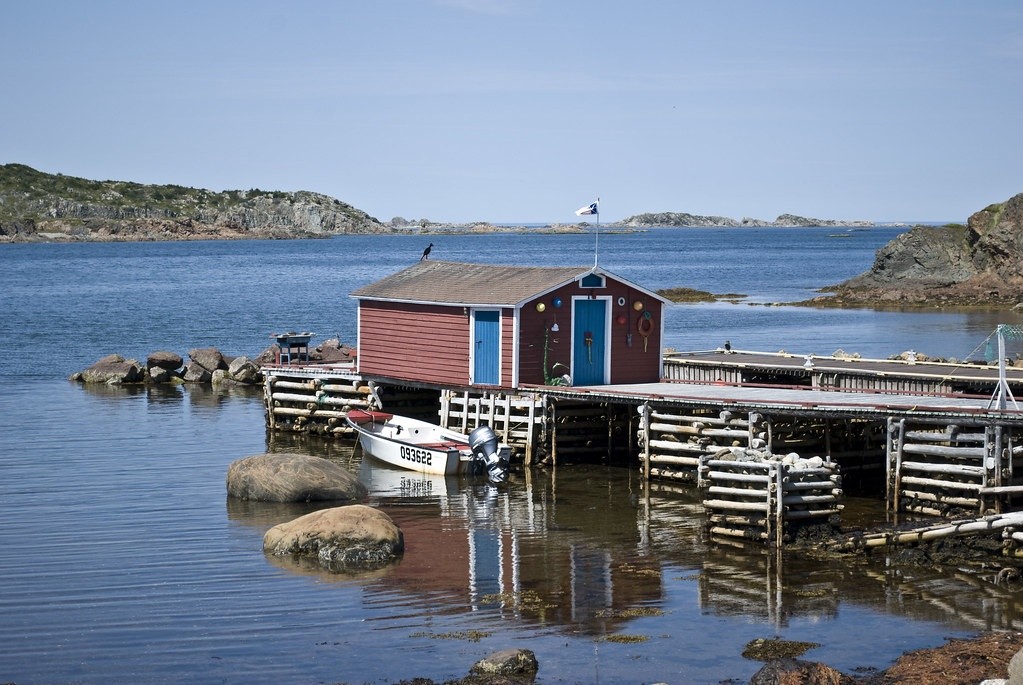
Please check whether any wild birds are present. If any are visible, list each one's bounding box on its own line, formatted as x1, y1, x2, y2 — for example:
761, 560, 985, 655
420, 243, 434, 261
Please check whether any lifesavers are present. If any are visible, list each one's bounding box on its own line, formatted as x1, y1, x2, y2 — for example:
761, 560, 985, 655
638, 316, 655, 337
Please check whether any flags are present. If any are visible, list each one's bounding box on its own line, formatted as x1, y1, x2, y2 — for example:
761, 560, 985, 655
575, 200, 598, 215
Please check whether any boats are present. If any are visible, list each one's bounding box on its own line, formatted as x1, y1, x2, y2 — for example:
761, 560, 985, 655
356, 452, 503, 511
344, 408, 514, 485
